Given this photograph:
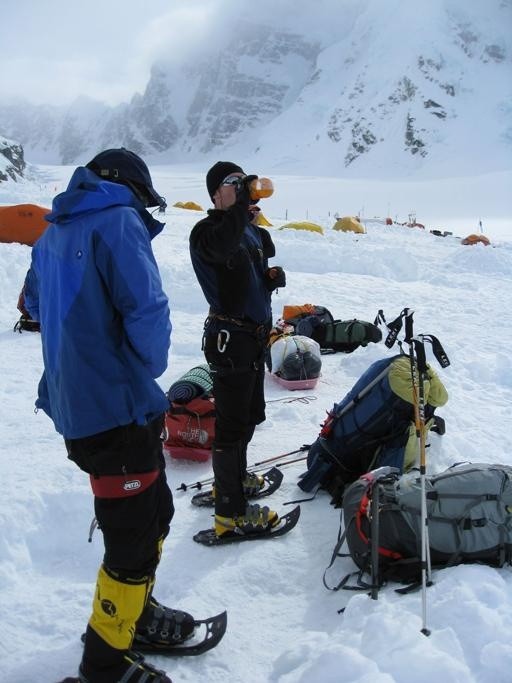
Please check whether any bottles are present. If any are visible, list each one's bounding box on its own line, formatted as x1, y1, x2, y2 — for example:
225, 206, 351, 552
248, 177, 274, 200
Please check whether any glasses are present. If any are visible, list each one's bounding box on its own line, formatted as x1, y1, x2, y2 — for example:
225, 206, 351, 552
220, 174, 247, 186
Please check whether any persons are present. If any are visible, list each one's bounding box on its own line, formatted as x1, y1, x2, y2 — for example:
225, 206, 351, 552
17, 146, 198, 682
187, 157, 288, 543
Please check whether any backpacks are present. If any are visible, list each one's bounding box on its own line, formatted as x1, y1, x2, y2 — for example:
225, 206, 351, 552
265, 303, 383, 374
303, 353, 447, 505
343, 460, 512, 579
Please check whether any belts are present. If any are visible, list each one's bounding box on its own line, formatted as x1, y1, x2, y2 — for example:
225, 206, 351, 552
208, 309, 273, 338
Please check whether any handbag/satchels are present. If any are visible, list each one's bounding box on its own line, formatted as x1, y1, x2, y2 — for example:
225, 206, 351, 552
163, 396, 216, 449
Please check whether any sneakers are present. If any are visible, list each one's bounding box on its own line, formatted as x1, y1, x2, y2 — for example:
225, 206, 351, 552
215, 508, 280, 539
136, 597, 195, 645
212, 473, 265, 499
79, 628, 172, 682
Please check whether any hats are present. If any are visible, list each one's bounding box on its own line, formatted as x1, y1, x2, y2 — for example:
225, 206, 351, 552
206, 160, 245, 202
88, 145, 166, 211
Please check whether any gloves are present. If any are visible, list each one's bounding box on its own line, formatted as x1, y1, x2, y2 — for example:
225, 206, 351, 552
266, 265, 287, 288
238, 173, 260, 205
